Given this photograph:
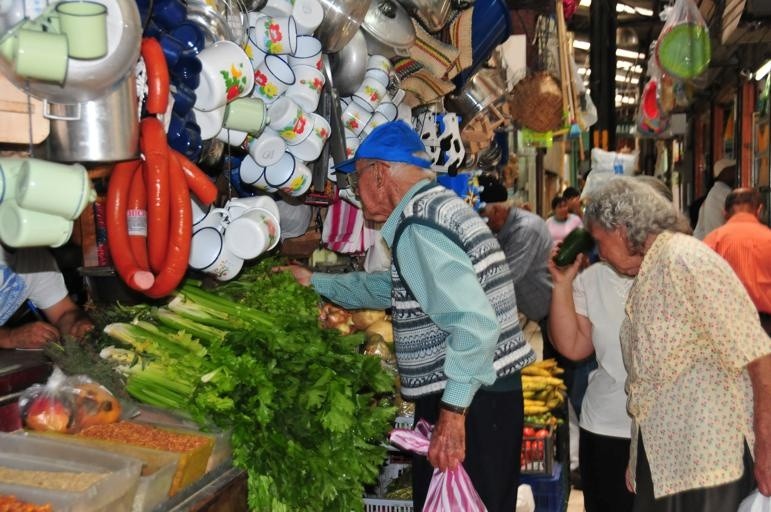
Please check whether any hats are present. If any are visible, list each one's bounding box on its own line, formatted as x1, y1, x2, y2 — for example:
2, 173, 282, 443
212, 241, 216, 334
330, 119, 435, 172
478, 175, 508, 203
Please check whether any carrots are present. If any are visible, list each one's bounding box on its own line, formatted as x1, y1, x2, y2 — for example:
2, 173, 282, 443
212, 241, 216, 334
522, 359, 567, 416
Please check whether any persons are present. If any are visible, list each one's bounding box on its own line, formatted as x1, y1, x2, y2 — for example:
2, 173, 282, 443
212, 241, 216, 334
0, 244, 94, 353
268, 119, 537, 512
471, 156, 771, 512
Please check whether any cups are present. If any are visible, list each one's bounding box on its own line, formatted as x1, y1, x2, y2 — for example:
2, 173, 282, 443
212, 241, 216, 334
338, 55, 403, 160
0, 155, 98, 251
188, 1, 333, 199
190, 194, 283, 285
0, 0, 110, 88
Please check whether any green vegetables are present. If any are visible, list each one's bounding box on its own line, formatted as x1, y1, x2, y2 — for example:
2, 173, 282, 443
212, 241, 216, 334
101, 286, 398, 512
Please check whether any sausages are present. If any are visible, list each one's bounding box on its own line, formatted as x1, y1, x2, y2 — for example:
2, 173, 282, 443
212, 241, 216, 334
106, 38, 218, 298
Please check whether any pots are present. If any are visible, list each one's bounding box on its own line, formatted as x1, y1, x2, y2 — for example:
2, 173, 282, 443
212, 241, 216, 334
0, 0, 144, 120
444, 65, 509, 136
360, 1, 418, 59
313, 0, 371, 99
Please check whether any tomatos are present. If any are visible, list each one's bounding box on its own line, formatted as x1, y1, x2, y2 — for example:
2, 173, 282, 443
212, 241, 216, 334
520, 427, 549, 465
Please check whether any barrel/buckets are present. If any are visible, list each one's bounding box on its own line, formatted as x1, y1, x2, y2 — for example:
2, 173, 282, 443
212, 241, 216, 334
48, 73, 142, 163
